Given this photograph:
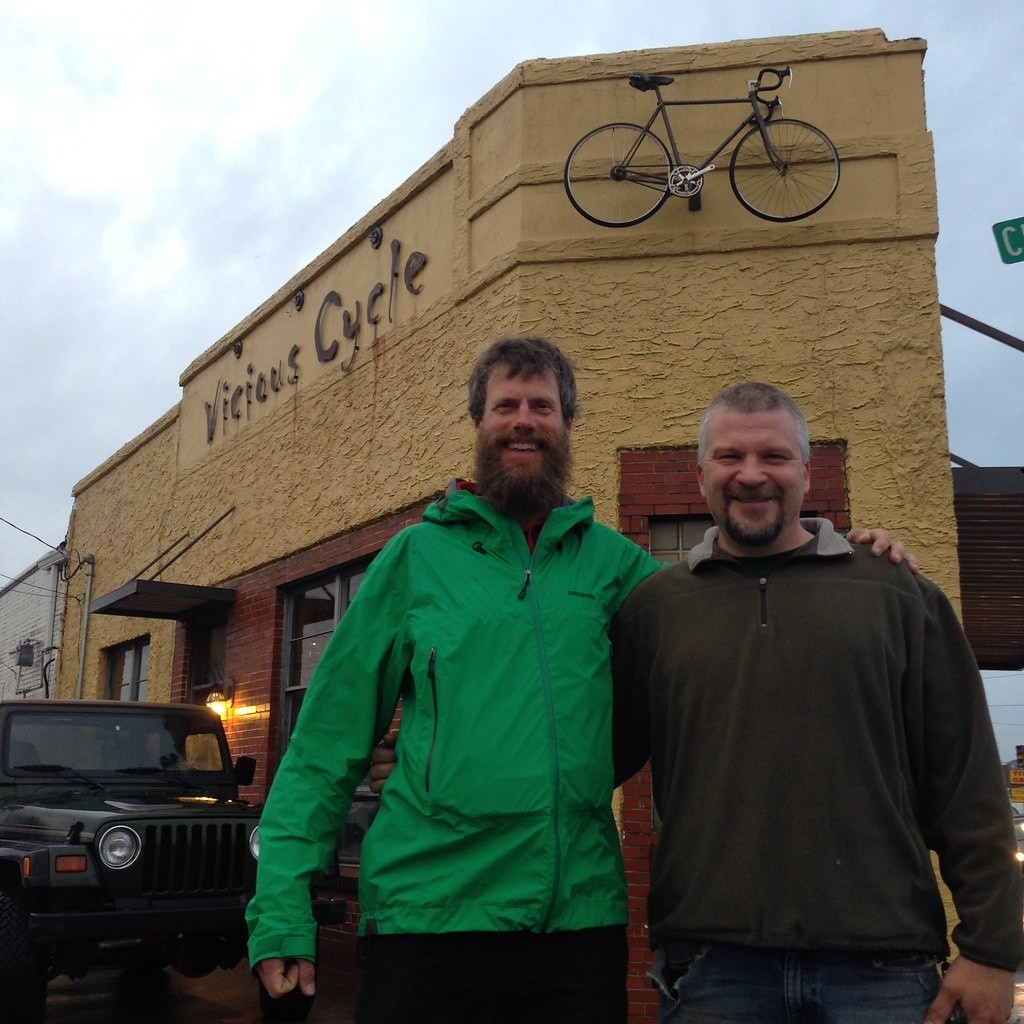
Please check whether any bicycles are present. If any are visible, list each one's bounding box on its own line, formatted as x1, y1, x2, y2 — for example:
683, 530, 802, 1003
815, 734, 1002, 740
564, 65, 840, 228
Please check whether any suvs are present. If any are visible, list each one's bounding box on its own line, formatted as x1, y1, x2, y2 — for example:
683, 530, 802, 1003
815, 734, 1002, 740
0, 698, 349, 1024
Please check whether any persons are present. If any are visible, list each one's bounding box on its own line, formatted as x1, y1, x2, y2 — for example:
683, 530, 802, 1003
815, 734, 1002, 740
251, 337, 928, 1023
370, 381, 1024, 1023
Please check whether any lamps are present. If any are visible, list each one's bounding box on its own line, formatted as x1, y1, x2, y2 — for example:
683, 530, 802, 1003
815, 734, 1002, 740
206, 676, 236, 715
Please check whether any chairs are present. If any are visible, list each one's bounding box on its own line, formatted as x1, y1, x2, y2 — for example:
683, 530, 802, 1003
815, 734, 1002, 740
8, 740, 41, 770
104, 731, 165, 768
